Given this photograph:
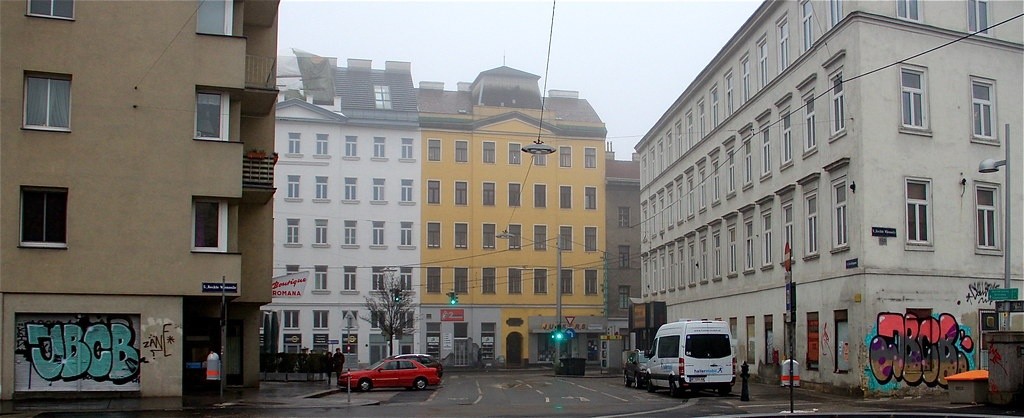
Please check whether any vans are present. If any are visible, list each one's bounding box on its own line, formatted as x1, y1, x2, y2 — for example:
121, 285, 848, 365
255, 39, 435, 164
646, 319, 735, 398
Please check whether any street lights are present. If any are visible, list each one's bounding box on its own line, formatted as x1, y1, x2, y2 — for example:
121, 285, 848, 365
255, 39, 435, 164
978, 123, 1012, 330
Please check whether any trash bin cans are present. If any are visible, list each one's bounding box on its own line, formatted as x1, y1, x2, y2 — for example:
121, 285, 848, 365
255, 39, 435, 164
559, 358, 587, 376
206, 352, 221, 380
781, 359, 801, 387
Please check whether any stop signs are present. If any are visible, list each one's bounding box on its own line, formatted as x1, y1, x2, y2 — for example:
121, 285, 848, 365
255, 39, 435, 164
784, 242, 791, 272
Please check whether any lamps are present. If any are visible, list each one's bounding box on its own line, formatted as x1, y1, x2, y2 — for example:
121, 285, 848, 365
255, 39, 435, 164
520, 140, 556, 155
496, 230, 515, 239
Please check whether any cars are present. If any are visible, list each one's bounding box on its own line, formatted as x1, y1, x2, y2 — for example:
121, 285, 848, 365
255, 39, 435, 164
337, 354, 443, 392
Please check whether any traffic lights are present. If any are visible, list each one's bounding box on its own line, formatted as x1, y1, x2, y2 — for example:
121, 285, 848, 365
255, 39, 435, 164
550, 330, 563, 342
450, 292, 459, 304
394, 289, 399, 302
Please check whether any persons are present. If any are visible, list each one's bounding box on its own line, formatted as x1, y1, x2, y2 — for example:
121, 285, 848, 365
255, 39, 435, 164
333, 348, 345, 388
323, 352, 335, 389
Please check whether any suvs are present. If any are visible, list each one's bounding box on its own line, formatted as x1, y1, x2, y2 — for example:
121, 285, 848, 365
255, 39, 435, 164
624, 349, 648, 389
